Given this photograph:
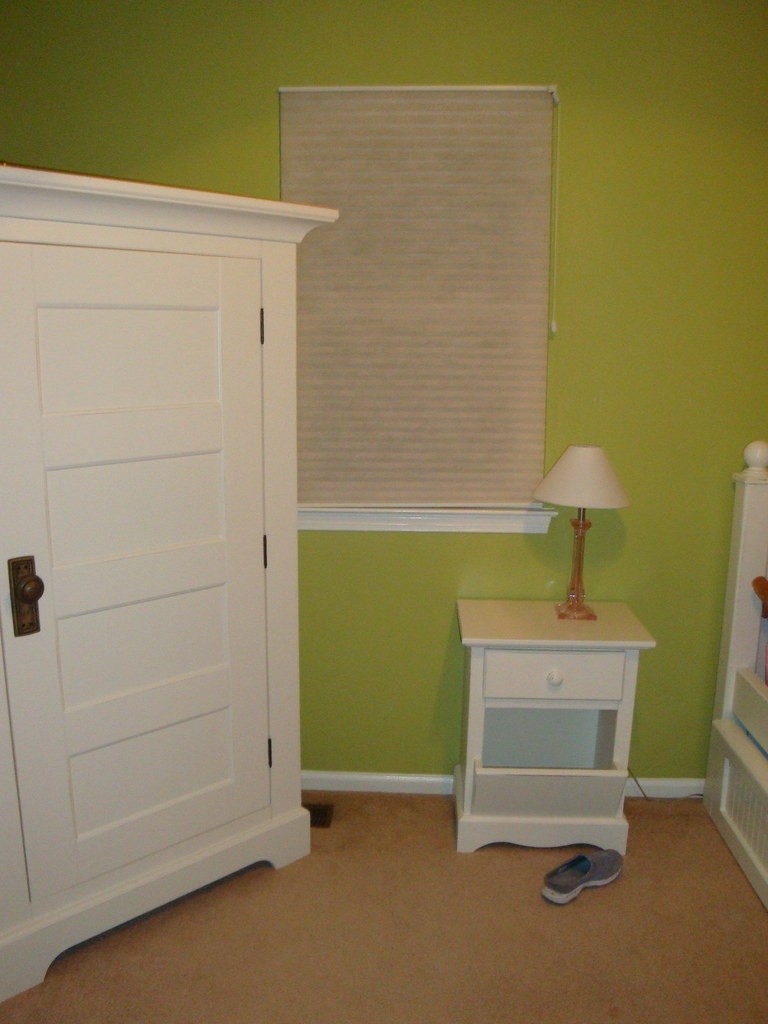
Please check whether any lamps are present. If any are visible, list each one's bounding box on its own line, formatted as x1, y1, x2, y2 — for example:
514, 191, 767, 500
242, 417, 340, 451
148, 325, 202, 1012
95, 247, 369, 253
533, 443, 631, 623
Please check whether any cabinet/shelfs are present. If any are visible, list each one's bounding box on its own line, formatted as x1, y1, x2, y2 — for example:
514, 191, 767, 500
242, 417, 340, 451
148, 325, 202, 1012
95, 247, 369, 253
1, 164, 338, 1006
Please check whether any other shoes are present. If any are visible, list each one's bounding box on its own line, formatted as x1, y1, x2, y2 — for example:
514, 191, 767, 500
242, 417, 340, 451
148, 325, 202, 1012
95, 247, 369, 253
540, 849, 622, 904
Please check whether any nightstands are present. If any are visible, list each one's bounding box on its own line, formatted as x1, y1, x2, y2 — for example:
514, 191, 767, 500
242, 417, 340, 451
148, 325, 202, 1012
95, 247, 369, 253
454, 598, 657, 855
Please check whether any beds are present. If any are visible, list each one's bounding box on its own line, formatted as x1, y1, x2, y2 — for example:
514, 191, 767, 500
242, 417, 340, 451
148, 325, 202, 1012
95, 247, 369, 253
702, 440, 768, 913
